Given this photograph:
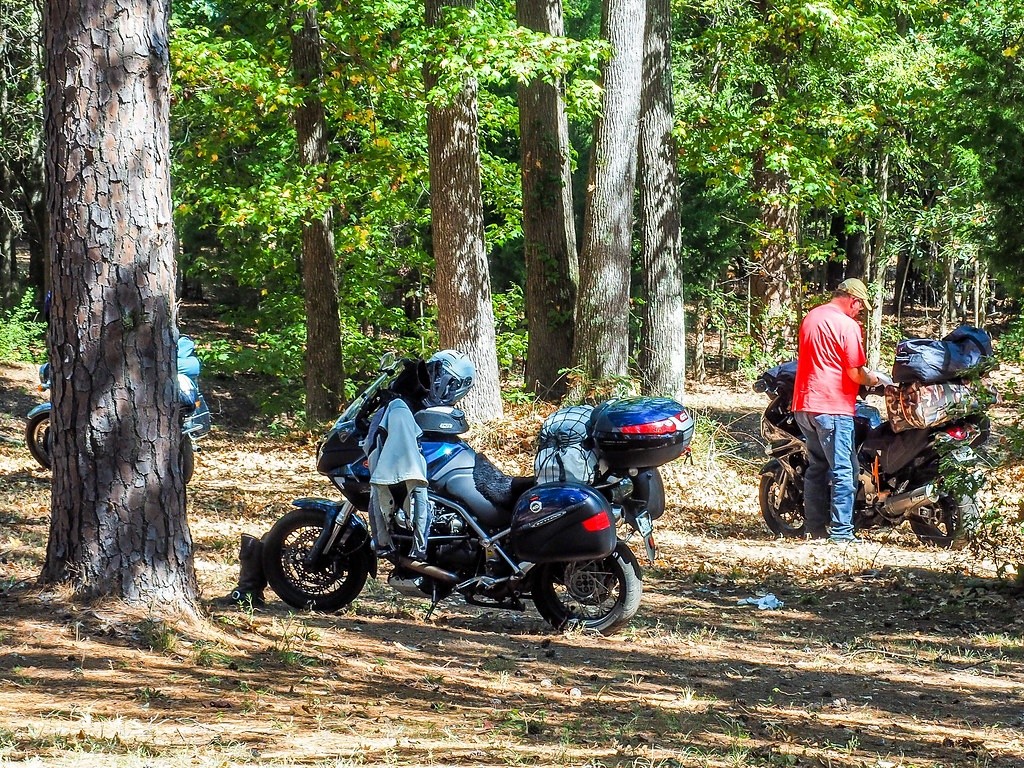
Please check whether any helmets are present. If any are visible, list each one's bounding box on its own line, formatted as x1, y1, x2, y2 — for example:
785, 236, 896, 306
421, 349, 475, 407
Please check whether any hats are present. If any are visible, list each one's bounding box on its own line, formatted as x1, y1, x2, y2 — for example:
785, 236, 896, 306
838, 278, 872, 311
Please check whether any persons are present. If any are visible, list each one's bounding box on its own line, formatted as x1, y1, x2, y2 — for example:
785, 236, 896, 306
793, 278, 884, 544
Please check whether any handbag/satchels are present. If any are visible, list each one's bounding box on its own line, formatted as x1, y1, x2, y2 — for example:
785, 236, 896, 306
892, 326, 992, 383
885, 378, 1003, 433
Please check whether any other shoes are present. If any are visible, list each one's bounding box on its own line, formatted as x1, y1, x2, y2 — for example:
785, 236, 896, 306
830, 535, 864, 543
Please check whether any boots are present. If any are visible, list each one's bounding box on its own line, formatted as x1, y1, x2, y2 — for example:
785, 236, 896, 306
224, 532, 268, 613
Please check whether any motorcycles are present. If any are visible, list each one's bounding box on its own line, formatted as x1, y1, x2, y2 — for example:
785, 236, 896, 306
21, 290, 194, 485
751, 361, 991, 551
263, 345, 694, 637
